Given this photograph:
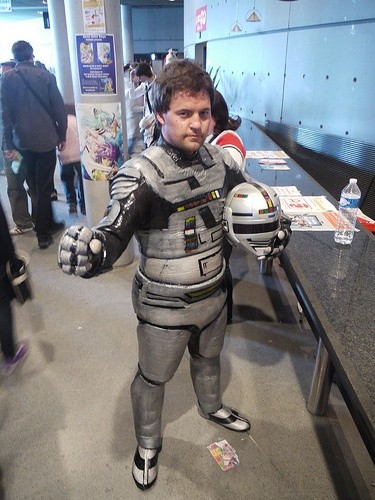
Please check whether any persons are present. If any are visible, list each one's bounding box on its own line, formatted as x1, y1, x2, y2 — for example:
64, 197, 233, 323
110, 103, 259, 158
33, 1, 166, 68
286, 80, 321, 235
0, 41, 67, 248
59, 61, 291, 489
0, 61, 247, 377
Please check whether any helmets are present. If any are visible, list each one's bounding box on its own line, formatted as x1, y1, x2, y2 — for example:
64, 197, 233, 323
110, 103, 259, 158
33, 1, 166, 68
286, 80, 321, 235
222, 182, 283, 254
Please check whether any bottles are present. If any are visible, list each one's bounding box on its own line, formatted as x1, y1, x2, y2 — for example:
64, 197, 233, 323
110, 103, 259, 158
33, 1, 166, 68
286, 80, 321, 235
333, 178, 362, 244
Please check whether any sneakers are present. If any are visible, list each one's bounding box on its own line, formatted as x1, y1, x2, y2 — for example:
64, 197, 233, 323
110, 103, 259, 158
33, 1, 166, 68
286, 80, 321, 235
8, 225, 33, 235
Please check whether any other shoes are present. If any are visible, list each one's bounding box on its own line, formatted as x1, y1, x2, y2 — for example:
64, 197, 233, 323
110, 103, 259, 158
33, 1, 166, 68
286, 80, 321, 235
51, 189, 60, 200
24, 229, 26, 230
80, 206, 87, 215
132, 444, 163, 490
51, 221, 65, 234
37, 235, 53, 249
4, 342, 28, 377
196, 407, 251, 432
68, 203, 77, 214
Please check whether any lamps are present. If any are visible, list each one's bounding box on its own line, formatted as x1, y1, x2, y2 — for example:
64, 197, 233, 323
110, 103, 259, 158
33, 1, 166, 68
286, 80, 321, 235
230, 0, 262, 33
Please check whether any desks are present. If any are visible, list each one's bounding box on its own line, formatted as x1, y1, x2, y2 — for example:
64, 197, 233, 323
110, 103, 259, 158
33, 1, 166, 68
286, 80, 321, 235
234, 117, 375, 466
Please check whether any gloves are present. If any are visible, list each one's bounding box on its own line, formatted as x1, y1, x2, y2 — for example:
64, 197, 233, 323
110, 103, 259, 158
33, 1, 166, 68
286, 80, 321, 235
258, 225, 293, 260
56, 225, 102, 277
